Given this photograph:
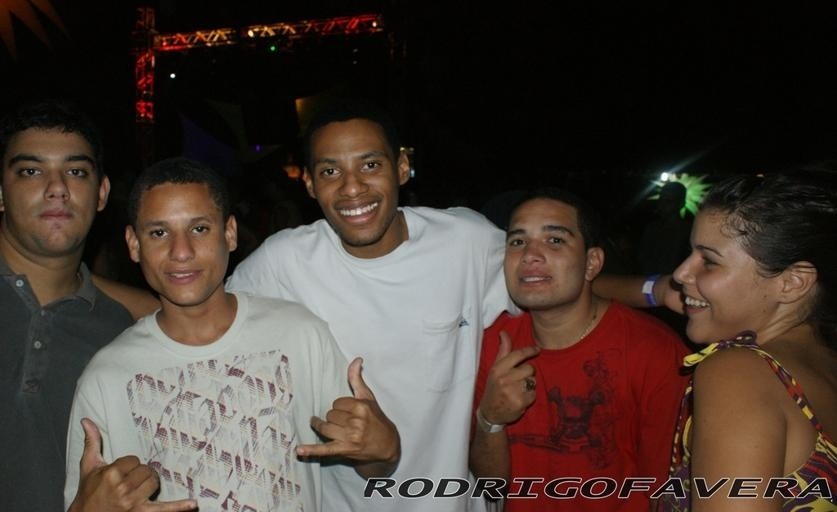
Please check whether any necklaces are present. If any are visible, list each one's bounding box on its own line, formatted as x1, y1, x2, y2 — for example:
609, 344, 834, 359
575, 294, 599, 343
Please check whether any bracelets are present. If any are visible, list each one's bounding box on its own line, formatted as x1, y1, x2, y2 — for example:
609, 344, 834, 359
640, 271, 664, 308
474, 407, 505, 434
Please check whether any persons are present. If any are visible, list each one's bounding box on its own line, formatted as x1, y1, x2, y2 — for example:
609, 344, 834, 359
592, 173, 700, 354
84, 140, 311, 297
87, 85, 689, 512
57, 154, 402, 512
0, 99, 144, 511
468, 184, 700, 509
648, 168, 836, 512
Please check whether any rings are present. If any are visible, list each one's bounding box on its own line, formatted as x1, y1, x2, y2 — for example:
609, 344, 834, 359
526, 378, 534, 391
